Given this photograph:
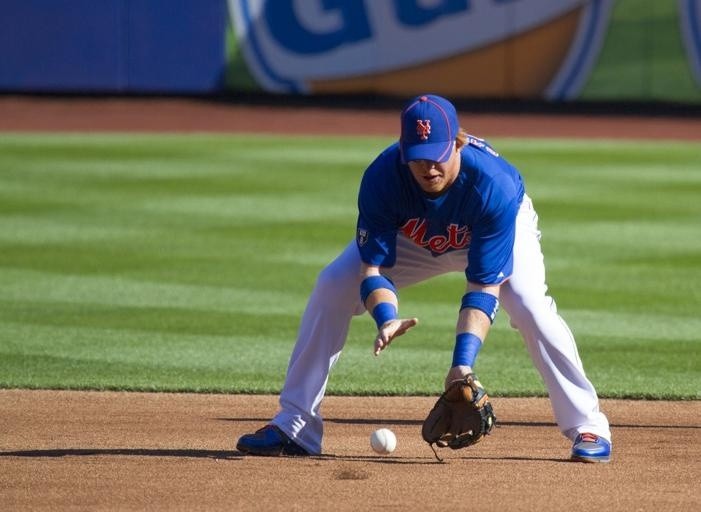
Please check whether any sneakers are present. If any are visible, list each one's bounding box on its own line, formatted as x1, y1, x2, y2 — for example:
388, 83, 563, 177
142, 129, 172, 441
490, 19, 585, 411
237, 425, 308, 456
570, 433, 610, 463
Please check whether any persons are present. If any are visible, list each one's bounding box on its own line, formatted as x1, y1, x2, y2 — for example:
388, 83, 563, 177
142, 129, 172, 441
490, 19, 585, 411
233, 91, 614, 466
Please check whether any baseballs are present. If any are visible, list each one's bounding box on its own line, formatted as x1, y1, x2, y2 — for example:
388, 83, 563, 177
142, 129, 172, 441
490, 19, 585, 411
371, 429, 397, 454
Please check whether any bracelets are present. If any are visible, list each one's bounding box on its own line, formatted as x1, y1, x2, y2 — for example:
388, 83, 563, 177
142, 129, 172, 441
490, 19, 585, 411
451, 331, 480, 369
366, 302, 400, 325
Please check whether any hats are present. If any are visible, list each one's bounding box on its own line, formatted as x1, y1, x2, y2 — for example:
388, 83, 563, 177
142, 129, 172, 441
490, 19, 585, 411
400, 94, 457, 160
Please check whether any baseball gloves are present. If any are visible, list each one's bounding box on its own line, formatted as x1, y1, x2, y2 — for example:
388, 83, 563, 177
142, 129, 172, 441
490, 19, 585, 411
422, 374, 495, 449
